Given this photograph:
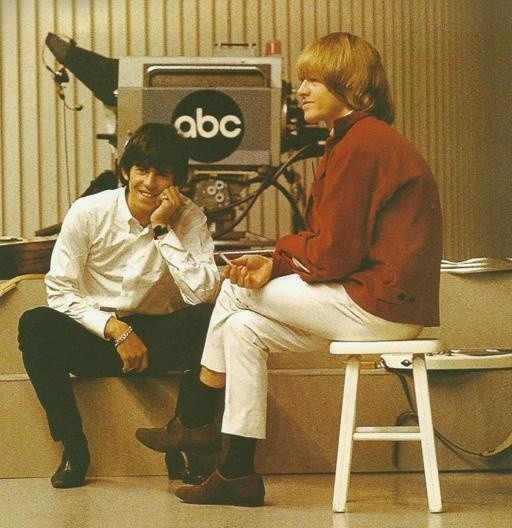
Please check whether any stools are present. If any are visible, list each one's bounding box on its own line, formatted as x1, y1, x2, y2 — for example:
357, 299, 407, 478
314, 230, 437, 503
324, 335, 444, 517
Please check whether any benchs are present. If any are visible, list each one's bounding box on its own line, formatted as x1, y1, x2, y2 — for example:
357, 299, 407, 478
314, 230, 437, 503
1, 263, 510, 480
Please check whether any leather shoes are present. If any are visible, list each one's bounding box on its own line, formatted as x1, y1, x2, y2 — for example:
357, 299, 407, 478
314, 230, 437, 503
51, 435, 91, 488
164, 448, 208, 486
135, 417, 223, 456
175, 468, 265, 507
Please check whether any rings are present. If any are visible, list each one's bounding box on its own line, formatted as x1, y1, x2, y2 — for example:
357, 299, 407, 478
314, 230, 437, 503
161, 196, 168, 200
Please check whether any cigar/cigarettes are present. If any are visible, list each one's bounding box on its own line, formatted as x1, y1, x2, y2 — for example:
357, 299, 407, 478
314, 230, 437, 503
218, 251, 234, 267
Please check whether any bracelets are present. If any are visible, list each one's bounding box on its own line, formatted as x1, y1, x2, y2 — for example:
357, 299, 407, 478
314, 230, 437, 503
114, 326, 133, 349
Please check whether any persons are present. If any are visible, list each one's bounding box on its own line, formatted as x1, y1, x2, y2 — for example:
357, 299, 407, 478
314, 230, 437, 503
135, 31, 443, 508
18, 122, 221, 490
